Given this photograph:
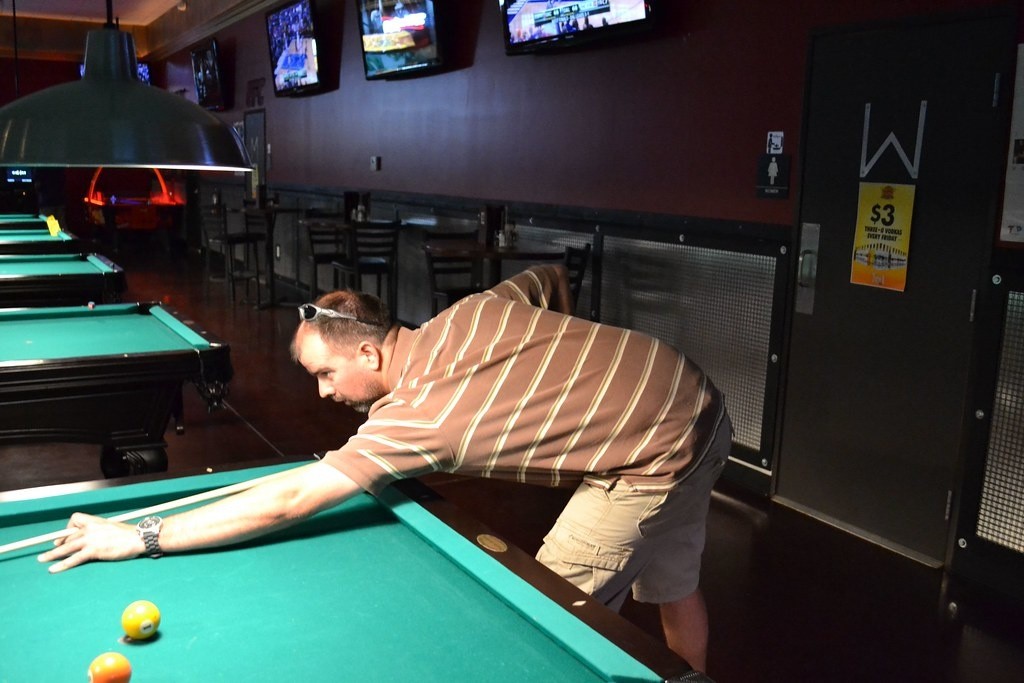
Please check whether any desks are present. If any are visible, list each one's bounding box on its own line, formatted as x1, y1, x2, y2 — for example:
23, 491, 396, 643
0, 227, 83, 254
214, 204, 318, 307
0, 213, 47, 230
1, 435, 721, 683
1, 249, 127, 306
0, 298, 231, 481
424, 239, 568, 295
300, 216, 393, 291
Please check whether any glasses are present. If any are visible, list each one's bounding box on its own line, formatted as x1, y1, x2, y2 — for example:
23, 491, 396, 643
297, 303, 384, 326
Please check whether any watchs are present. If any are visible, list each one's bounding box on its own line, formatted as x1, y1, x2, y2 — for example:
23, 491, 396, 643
137, 516, 164, 560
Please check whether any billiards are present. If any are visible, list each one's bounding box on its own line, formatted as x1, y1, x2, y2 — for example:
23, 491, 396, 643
87, 651, 132, 683
122, 598, 162, 642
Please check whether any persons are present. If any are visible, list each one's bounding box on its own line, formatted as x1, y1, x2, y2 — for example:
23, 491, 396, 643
37, 261, 734, 675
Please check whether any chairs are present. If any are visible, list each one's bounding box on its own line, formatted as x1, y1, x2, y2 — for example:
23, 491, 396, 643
201, 189, 592, 330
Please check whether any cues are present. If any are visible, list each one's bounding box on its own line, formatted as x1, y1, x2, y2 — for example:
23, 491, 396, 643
0, 462, 315, 556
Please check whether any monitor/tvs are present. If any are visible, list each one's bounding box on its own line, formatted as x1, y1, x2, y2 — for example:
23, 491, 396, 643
132, 0, 654, 113
